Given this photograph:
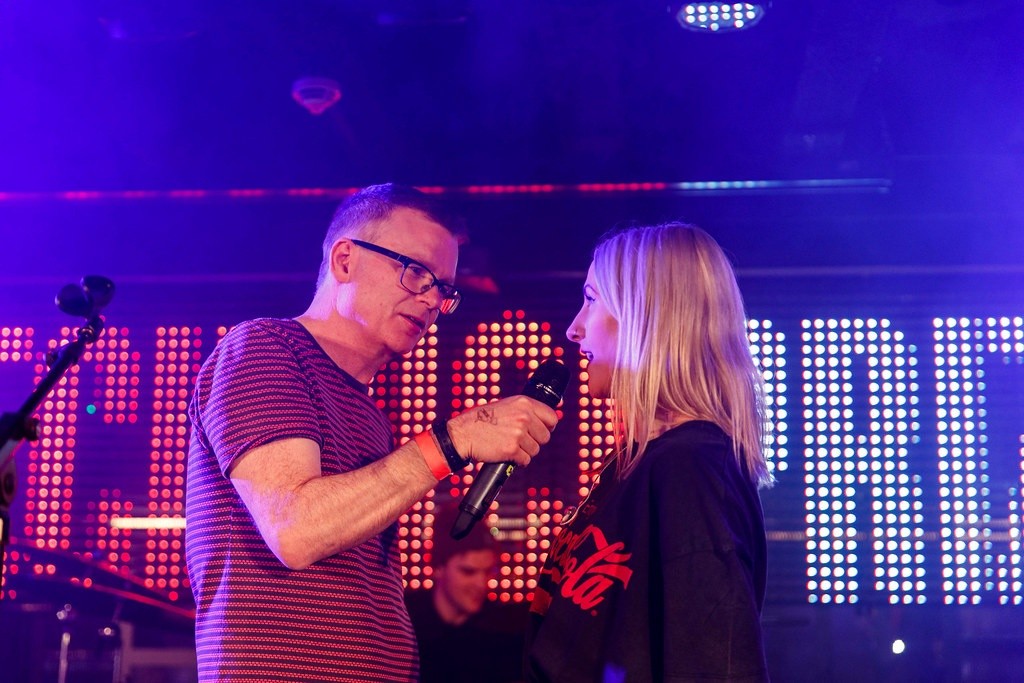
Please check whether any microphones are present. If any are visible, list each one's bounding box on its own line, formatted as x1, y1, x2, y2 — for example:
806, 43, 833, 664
450, 359, 569, 542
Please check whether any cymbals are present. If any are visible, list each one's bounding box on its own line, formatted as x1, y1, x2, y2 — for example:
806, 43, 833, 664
6, 542, 169, 597
24, 574, 195, 621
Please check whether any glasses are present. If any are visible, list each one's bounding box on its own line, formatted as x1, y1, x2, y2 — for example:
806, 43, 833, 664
351, 238, 462, 315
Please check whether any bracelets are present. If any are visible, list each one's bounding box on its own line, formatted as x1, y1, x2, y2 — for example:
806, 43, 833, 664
432, 414, 469, 473
414, 430, 452, 481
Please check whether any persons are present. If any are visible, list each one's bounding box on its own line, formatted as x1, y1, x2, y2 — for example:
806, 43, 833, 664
184, 183, 559, 683
528, 220, 771, 683
